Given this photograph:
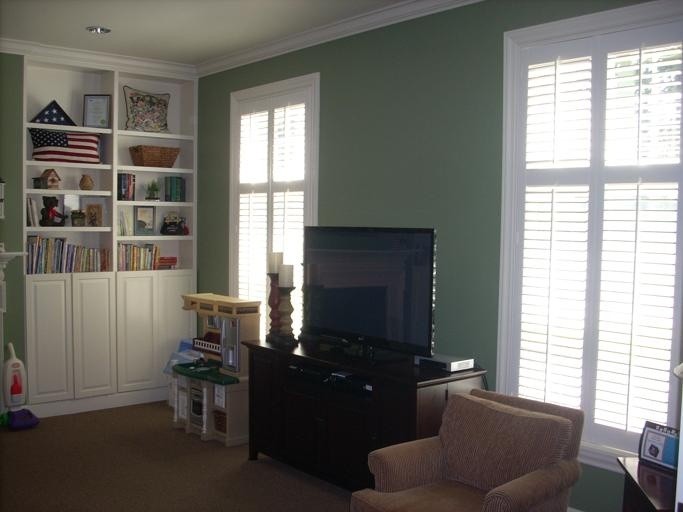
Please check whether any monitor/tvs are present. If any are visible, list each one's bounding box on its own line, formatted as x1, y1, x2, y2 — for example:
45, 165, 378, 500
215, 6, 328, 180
302, 225, 437, 357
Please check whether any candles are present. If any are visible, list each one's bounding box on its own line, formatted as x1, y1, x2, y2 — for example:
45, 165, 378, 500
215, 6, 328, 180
268, 252, 282, 274
277, 263, 293, 287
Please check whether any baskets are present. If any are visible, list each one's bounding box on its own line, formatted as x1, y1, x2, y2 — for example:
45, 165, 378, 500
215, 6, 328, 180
129, 145, 180, 167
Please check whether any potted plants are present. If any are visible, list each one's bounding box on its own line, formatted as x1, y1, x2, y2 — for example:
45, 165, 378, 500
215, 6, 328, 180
142, 178, 161, 201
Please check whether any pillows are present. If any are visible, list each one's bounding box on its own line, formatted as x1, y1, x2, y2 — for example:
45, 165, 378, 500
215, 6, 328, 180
440, 393, 573, 489
29, 128, 102, 163
124, 85, 172, 133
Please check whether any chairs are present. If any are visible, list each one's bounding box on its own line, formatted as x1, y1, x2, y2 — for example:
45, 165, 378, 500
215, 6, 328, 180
350, 389, 584, 512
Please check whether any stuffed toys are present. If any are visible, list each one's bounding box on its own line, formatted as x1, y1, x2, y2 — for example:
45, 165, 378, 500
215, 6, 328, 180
39, 196, 67, 226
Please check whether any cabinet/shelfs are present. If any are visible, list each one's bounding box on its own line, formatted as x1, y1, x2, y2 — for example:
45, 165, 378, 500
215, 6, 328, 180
241, 337, 488, 492
173, 293, 260, 448
21, 48, 198, 417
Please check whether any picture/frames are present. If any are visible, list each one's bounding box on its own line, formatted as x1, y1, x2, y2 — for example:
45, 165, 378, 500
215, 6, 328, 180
83, 94, 111, 128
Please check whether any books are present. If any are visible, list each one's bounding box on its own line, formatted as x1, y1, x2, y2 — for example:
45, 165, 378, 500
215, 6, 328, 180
164, 175, 186, 202
26, 235, 110, 275
115, 242, 179, 271
117, 173, 137, 201
117, 210, 130, 236
27, 199, 42, 226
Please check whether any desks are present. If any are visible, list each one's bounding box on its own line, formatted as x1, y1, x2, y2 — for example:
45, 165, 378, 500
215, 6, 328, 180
617, 456, 675, 512
